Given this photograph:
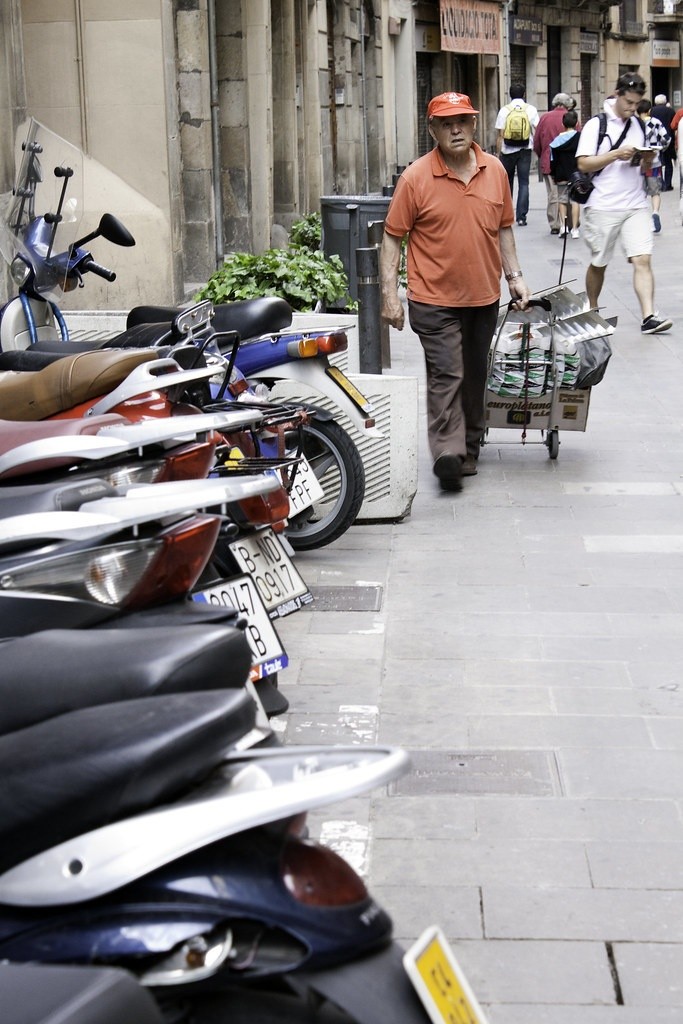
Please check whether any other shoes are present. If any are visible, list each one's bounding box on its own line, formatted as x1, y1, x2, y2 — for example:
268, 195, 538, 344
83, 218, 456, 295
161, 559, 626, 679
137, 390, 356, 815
551, 229, 559, 234
462, 453, 479, 475
569, 223, 580, 231
433, 450, 464, 492
661, 186, 673, 192
518, 219, 527, 226
652, 212, 662, 233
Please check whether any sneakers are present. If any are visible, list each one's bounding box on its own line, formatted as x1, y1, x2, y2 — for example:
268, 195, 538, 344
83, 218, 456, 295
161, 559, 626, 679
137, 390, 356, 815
640, 310, 673, 334
571, 228, 579, 239
559, 225, 569, 238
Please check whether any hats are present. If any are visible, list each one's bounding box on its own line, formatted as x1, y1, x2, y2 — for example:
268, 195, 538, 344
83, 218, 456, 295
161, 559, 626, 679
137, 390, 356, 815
426, 91, 479, 124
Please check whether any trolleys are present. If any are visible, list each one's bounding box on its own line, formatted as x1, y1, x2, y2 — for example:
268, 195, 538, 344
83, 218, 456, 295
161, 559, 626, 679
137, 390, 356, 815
476, 293, 563, 459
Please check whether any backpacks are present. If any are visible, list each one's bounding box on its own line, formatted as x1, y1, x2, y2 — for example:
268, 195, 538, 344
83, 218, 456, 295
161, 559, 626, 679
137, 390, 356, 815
503, 103, 530, 147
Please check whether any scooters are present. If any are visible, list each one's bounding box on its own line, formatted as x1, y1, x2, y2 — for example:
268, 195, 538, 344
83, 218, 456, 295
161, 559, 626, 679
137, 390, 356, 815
0, 138, 493, 1024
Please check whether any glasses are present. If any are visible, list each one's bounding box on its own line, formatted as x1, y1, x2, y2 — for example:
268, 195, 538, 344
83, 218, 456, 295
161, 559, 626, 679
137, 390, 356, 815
616, 81, 646, 90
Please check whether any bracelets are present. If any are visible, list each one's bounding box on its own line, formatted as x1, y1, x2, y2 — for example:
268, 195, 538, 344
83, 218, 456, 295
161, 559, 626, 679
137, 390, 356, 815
506, 271, 522, 281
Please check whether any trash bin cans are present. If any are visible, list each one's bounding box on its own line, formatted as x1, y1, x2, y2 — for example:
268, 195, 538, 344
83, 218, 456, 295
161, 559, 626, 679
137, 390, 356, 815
320, 195, 392, 314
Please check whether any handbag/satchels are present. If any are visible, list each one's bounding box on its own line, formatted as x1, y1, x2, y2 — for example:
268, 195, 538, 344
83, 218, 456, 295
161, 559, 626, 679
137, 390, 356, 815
567, 171, 595, 204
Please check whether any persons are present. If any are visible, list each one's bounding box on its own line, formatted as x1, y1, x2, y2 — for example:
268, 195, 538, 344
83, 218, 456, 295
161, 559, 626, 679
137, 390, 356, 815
574, 73, 672, 334
496, 83, 539, 225
378, 92, 532, 492
636, 94, 683, 232
533, 93, 580, 240
